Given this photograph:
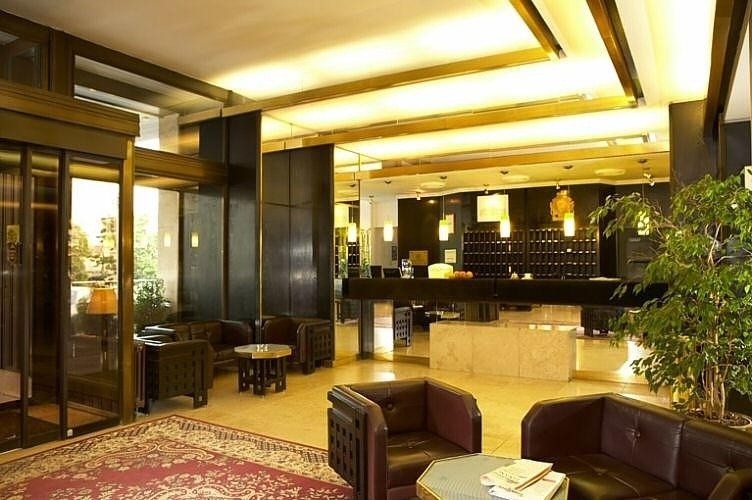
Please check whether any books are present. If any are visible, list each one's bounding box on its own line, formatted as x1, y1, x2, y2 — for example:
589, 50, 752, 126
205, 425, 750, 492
480, 457, 554, 491
488, 471, 567, 500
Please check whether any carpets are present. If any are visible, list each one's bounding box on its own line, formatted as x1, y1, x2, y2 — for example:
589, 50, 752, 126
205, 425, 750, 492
1, 418, 356, 499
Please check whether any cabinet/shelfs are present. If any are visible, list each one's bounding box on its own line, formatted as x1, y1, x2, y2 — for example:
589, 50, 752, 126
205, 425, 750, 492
463, 227, 602, 278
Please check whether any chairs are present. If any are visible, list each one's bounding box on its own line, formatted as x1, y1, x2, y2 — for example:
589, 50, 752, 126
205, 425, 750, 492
263, 317, 333, 374
326, 376, 481, 500
142, 336, 212, 415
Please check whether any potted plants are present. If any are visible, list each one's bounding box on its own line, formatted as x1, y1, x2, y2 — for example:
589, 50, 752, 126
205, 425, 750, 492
589, 171, 751, 431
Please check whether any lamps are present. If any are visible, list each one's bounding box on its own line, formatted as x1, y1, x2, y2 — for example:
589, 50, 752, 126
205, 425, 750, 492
383, 180, 394, 241
439, 176, 448, 241
347, 185, 357, 242
85, 288, 118, 372
500, 172, 510, 238
564, 166, 575, 236
637, 160, 650, 235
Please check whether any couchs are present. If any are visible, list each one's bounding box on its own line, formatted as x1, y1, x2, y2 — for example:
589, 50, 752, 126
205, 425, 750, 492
522, 392, 751, 499
144, 319, 253, 369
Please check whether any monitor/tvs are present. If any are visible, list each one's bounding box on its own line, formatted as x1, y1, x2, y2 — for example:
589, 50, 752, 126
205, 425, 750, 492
383, 268, 401, 278
370, 265, 382, 279
